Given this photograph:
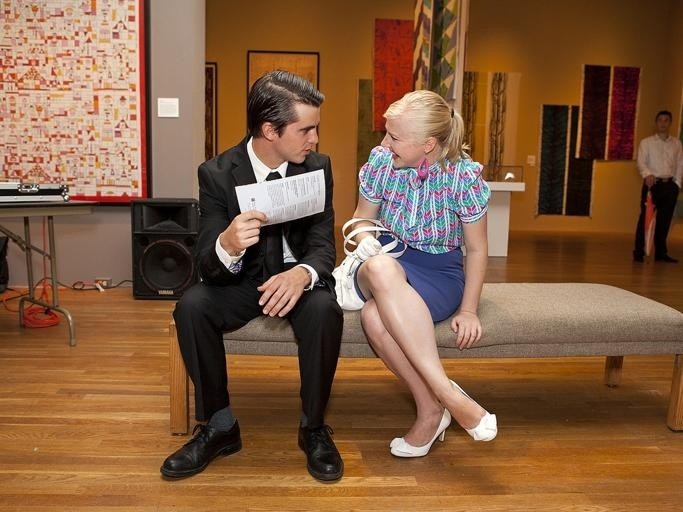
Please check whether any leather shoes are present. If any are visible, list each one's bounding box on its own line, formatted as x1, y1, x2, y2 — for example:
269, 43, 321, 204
654, 255, 679, 263
298, 421, 343, 481
160, 419, 242, 478
634, 256, 644, 262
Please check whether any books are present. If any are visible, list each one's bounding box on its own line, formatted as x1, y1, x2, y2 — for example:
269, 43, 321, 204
233, 168, 328, 228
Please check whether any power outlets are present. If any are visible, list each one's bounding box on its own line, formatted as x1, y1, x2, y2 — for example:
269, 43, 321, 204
94, 278, 112, 289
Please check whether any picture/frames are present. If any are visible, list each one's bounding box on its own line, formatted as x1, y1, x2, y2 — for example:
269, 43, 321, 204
205, 60, 217, 165
246, 50, 320, 155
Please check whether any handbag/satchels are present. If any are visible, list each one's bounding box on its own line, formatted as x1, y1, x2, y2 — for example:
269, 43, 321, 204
331, 217, 408, 311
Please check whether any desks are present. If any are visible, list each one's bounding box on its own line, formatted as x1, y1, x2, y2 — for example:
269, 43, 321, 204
0, 201, 97, 346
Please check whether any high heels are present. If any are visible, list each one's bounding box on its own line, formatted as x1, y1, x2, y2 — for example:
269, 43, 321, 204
446, 380, 497, 441
391, 408, 451, 457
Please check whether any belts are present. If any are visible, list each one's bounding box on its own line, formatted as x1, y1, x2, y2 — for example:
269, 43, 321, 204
656, 178, 673, 183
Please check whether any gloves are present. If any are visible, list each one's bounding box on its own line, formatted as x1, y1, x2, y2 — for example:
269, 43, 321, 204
357, 236, 382, 261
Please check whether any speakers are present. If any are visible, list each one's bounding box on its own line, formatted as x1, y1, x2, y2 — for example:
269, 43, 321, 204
130, 198, 200, 300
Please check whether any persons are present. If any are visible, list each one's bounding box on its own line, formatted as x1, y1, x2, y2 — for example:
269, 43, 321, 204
631, 110, 683, 264
347, 87, 498, 460
160, 66, 345, 484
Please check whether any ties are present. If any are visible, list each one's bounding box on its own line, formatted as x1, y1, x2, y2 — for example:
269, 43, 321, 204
259, 172, 283, 283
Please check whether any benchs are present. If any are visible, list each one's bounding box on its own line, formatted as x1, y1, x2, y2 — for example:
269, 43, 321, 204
167, 278, 683, 434
